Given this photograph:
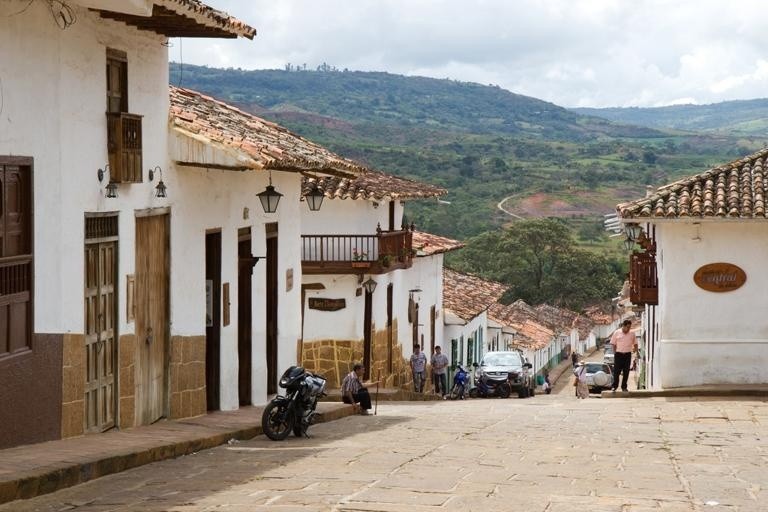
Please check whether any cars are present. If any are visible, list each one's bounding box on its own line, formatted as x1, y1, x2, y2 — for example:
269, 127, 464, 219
473, 351, 537, 398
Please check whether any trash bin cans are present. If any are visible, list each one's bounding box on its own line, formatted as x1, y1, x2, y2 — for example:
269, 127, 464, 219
537, 375, 544, 385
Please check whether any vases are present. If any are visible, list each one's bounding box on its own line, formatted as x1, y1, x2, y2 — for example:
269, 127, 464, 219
352, 262, 370, 268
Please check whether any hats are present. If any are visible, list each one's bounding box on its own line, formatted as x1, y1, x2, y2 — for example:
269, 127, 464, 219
578, 361, 586, 366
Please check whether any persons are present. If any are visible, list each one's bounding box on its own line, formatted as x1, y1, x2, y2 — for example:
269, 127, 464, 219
574, 355, 579, 368
609, 320, 641, 392
572, 352, 576, 366
341, 364, 382, 416
409, 344, 428, 393
574, 360, 590, 399
431, 346, 449, 400
545, 375, 551, 394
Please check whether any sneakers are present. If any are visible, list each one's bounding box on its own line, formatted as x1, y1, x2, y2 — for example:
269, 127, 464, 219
611, 387, 628, 391
361, 409, 373, 415
435, 392, 447, 400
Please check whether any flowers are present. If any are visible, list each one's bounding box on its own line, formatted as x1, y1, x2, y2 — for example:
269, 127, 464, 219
352, 247, 368, 262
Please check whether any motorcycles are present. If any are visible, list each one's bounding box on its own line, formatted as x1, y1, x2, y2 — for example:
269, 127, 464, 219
262, 366, 327, 441
469, 373, 511, 398
450, 362, 472, 400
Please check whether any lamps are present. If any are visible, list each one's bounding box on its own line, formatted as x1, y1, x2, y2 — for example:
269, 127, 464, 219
98, 165, 117, 198
362, 274, 377, 294
255, 171, 283, 213
149, 166, 167, 197
624, 223, 648, 250
303, 170, 327, 210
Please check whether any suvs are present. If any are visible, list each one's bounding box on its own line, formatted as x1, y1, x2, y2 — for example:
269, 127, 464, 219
573, 362, 614, 396
